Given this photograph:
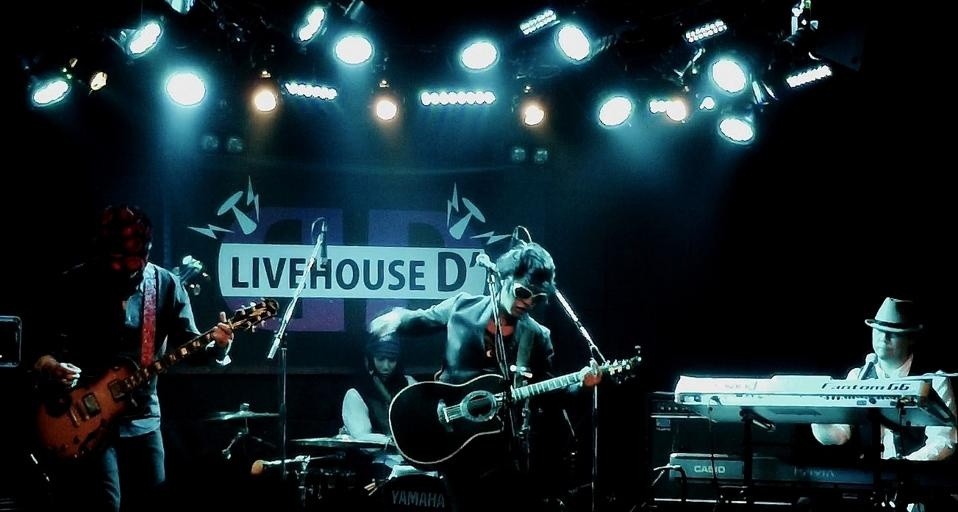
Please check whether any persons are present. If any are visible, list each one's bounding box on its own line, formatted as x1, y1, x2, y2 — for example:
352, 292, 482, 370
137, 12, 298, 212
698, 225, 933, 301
19, 204, 234, 512
365, 240, 603, 511
341, 333, 444, 478
793, 296, 958, 512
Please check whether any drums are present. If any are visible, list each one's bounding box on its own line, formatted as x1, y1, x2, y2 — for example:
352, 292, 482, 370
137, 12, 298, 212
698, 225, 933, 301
256, 456, 376, 512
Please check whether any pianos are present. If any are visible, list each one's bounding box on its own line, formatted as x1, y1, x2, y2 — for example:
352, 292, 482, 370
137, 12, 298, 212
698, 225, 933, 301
669, 372, 953, 501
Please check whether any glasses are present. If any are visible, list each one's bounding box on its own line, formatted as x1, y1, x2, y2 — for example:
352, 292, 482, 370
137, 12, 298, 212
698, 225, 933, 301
505, 274, 549, 307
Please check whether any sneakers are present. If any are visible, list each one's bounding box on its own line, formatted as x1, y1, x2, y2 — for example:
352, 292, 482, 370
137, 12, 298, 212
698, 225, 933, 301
864, 297, 923, 334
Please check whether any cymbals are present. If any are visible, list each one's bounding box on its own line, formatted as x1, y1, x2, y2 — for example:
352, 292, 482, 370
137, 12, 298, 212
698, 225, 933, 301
202, 409, 279, 423
291, 432, 394, 449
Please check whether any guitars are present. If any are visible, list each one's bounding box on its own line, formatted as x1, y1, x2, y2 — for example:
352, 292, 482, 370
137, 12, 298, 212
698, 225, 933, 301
388, 346, 644, 473
25, 296, 279, 478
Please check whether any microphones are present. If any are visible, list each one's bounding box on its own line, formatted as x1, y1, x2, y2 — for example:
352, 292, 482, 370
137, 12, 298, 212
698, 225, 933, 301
857, 353, 878, 380
320, 220, 328, 268
509, 227, 518, 250
476, 253, 499, 272
